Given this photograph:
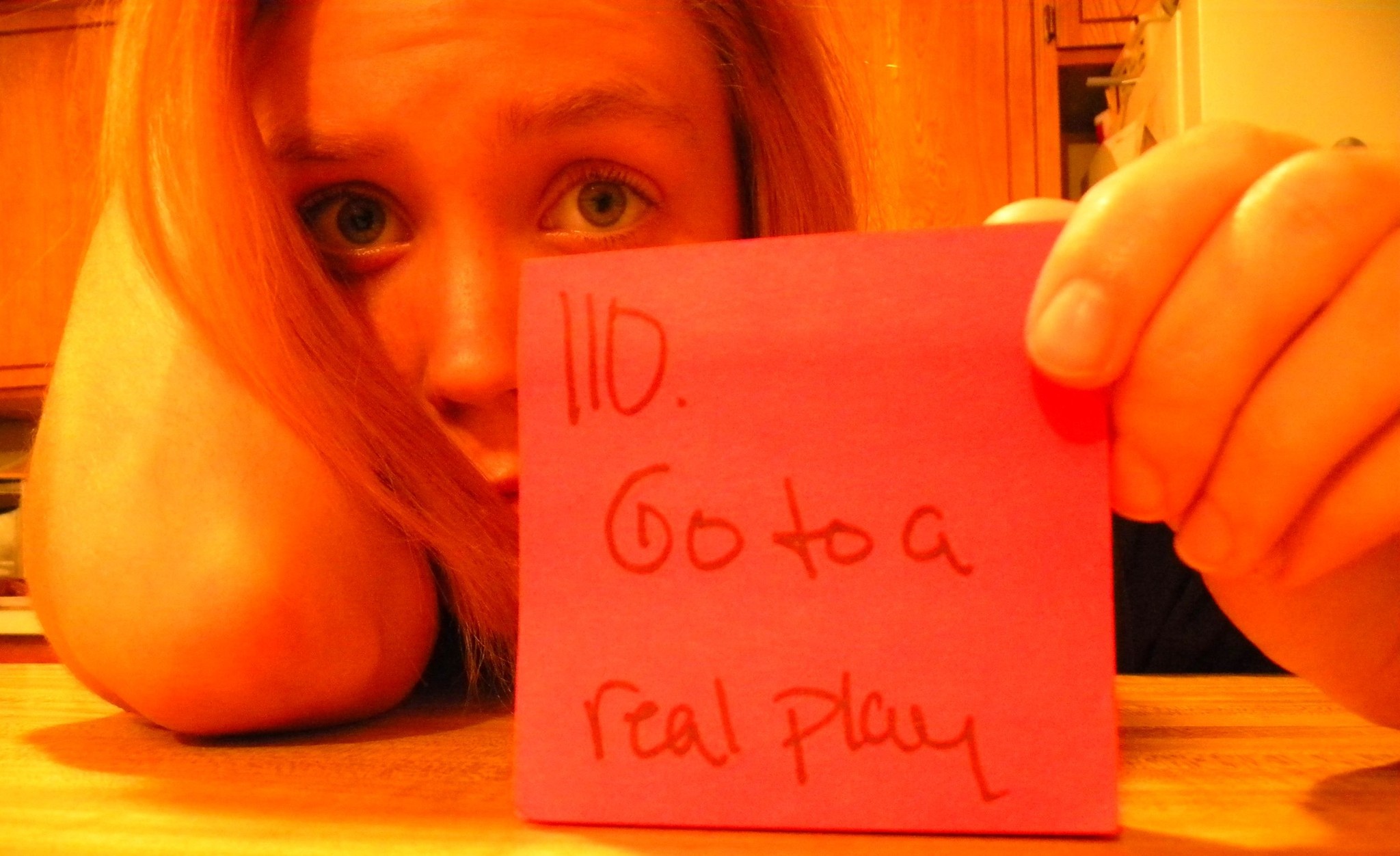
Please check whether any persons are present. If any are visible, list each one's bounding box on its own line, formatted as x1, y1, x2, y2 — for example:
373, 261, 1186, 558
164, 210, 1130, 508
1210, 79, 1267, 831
16, 0, 1400, 739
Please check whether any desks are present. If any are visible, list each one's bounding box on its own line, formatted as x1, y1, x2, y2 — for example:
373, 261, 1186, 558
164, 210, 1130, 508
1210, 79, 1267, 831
0, 657, 1399, 856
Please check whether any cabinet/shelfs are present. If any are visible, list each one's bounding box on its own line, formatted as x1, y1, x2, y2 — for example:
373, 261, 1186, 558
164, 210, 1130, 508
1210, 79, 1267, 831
0, 1, 1165, 424
